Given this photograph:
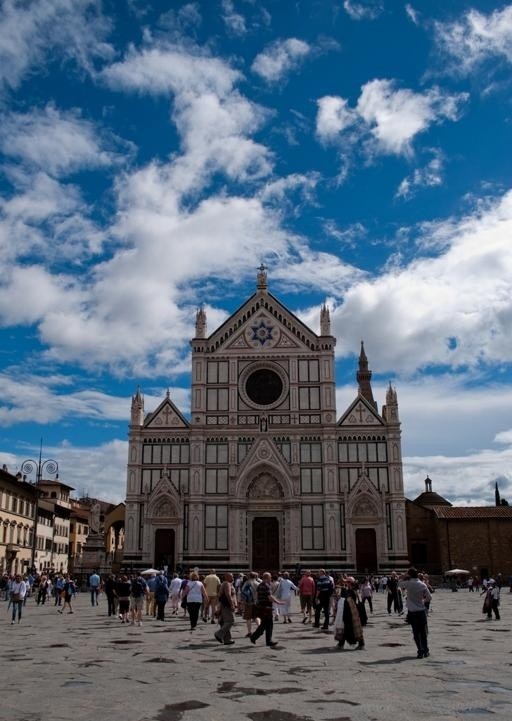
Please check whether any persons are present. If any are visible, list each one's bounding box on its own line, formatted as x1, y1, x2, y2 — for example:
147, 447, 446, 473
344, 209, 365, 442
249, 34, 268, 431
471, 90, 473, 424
370, 569, 435, 658
1, 567, 80, 625
89, 568, 301, 646
297, 568, 375, 650
443, 573, 507, 621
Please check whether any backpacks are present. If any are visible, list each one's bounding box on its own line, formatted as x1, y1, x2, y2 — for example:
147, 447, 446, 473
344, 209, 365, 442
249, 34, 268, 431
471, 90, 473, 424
133, 578, 142, 598
241, 585, 254, 602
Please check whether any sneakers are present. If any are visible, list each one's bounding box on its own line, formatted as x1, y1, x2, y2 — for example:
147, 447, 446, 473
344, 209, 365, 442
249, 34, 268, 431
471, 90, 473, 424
266, 641, 277, 646
224, 640, 235, 645
250, 636, 255, 644
214, 634, 222, 643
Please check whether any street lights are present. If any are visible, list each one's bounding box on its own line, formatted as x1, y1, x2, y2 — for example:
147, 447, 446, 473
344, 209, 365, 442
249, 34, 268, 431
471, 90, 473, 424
19, 435, 61, 577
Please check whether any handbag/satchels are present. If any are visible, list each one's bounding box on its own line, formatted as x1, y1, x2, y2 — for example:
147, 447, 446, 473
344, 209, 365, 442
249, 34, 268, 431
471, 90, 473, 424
219, 582, 230, 607
11, 591, 20, 602
181, 595, 187, 608
